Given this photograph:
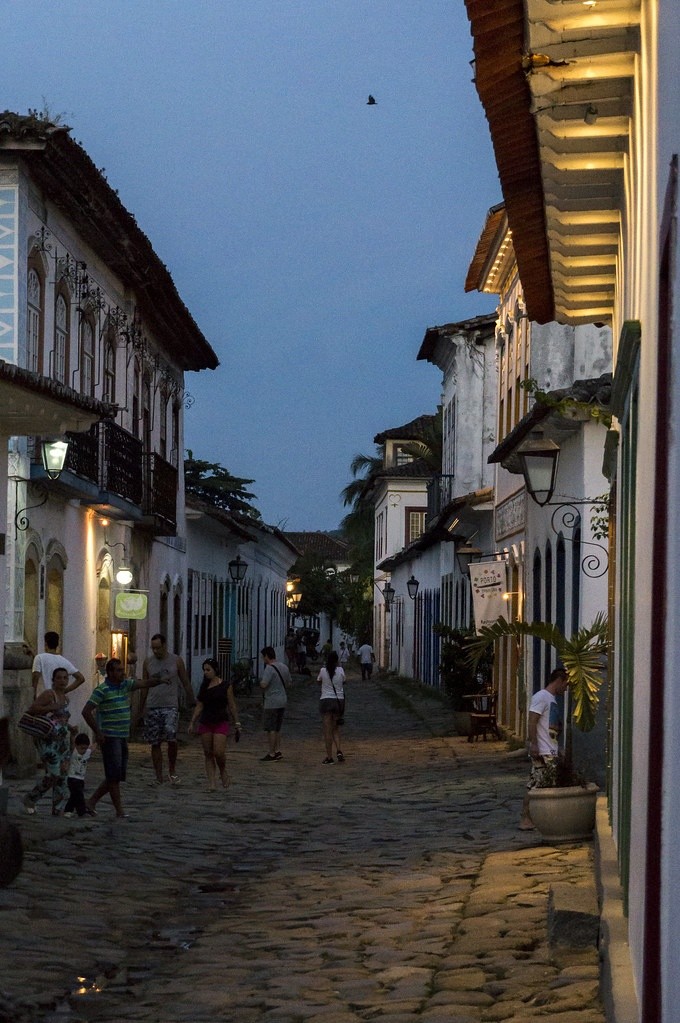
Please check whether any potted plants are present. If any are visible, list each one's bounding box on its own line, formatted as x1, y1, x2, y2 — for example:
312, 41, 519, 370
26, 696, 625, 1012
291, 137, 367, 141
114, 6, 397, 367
462, 612, 611, 842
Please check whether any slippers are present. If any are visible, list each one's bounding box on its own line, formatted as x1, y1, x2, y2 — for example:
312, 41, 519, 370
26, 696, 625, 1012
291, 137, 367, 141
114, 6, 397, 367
223, 775, 230, 787
205, 788, 216, 792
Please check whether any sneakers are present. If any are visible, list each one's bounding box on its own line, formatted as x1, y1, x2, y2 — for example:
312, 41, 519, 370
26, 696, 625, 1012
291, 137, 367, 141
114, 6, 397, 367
321, 757, 335, 765
337, 751, 345, 762
260, 754, 279, 762
276, 751, 283, 758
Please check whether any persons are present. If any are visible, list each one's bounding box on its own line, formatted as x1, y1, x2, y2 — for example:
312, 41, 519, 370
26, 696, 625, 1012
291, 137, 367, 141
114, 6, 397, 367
357, 641, 376, 681
32, 631, 86, 767
64, 727, 97, 819
517, 669, 568, 830
284, 627, 350, 685
316, 651, 346, 765
136, 633, 196, 786
81, 658, 173, 819
259, 646, 292, 762
188, 657, 242, 791
19, 667, 72, 818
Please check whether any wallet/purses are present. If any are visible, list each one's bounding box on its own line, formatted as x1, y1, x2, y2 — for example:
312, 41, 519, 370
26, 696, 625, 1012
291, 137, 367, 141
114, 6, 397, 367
235, 729, 241, 742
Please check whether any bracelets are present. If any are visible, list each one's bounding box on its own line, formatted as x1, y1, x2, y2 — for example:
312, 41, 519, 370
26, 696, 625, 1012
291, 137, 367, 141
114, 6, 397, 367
190, 720, 196, 723
235, 722, 241, 726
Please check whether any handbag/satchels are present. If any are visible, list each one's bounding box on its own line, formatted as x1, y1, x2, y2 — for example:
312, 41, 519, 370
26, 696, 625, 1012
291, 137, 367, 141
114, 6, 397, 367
339, 698, 345, 714
17, 690, 58, 739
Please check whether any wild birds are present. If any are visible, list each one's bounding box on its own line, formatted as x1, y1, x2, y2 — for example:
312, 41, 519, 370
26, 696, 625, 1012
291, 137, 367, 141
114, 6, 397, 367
366, 94, 378, 105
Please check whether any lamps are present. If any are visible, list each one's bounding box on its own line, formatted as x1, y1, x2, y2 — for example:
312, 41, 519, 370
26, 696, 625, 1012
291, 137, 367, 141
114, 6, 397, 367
518, 425, 608, 506
406, 576, 432, 602
382, 582, 401, 605
456, 541, 483, 578
222, 555, 248, 584
14, 435, 71, 533
285, 589, 303, 605
105, 538, 132, 584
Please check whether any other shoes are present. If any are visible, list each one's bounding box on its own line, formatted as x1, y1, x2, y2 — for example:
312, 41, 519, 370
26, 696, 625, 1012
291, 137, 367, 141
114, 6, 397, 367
170, 775, 180, 784
64, 811, 72, 818
148, 781, 162, 786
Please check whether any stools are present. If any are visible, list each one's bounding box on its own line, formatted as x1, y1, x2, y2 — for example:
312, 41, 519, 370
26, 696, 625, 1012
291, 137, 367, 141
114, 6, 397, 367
470, 713, 500, 742
